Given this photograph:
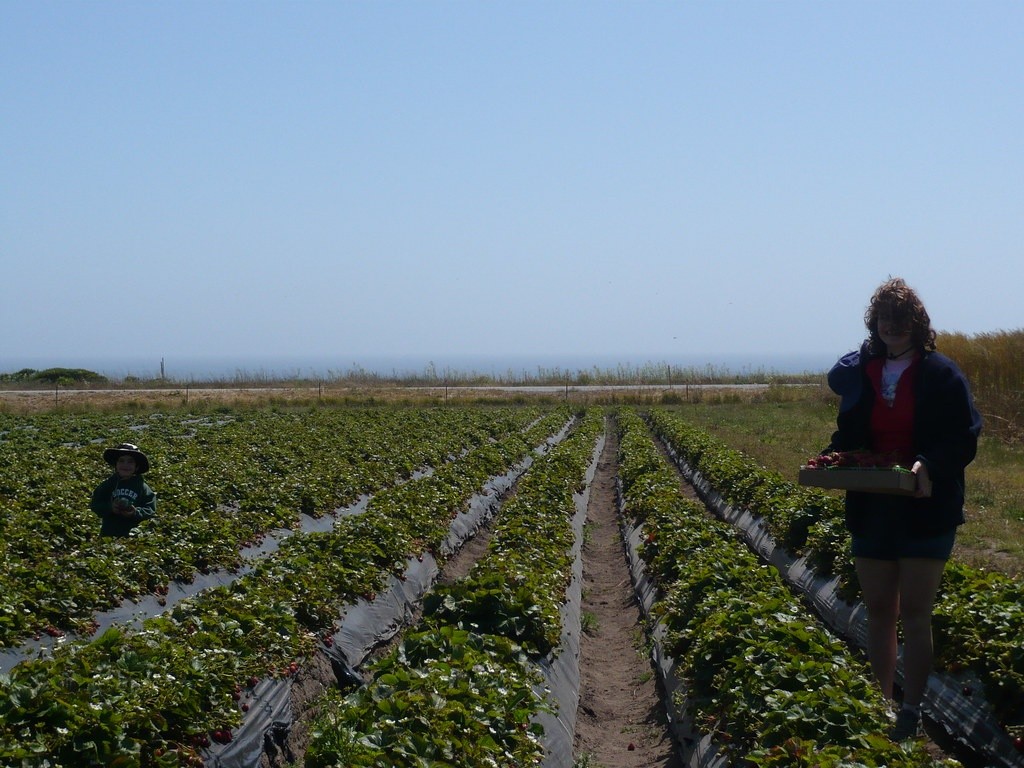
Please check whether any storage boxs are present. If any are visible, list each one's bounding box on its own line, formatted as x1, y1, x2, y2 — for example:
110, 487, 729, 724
797, 470, 932, 499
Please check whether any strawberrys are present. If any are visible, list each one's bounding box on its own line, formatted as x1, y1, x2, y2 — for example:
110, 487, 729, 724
804, 450, 911, 470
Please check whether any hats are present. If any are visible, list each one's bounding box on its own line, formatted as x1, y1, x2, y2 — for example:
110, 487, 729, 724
102, 443, 150, 475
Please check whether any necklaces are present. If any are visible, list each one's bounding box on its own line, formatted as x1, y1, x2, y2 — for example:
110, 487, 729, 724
888, 346, 914, 360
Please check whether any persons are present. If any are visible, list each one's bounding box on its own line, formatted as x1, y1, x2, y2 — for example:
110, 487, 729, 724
812, 278, 982, 742
88, 443, 156, 538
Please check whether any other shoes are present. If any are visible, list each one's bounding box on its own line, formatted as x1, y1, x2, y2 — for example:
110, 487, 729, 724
889, 711, 923, 742
